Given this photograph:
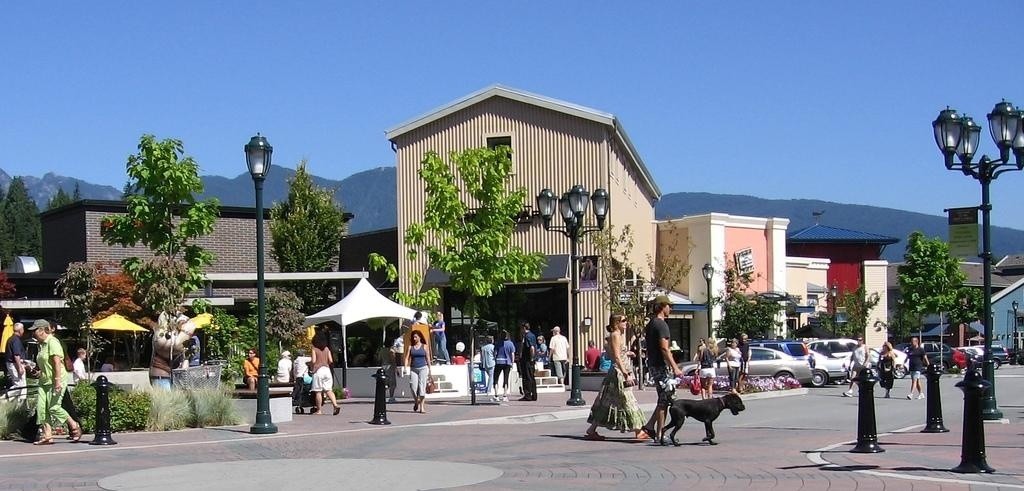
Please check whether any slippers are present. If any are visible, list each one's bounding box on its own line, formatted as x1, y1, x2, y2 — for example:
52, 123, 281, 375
312, 410, 322, 414
584, 431, 605, 441
333, 406, 341, 415
637, 426, 656, 441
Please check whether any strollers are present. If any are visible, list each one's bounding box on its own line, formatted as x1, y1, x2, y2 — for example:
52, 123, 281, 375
292, 368, 326, 415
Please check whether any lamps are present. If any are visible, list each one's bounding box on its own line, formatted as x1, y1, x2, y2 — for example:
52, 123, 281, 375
583, 316, 591, 333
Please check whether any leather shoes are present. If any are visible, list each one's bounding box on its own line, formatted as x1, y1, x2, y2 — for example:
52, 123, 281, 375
413, 402, 426, 413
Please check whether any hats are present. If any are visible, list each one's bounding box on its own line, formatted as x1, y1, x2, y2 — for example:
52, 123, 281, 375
551, 326, 561, 332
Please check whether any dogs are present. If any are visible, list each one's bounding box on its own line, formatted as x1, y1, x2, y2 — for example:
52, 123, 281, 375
659, 384, 746, 447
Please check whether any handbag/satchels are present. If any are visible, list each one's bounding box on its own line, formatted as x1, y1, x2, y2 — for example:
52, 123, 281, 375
479, 363, 486, 371
689, 373, 701, 395
425, 377, 436, 394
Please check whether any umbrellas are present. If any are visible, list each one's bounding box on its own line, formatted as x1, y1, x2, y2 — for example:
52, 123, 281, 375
192, 312, 239, 353
79, 312, 150, 362
0, 314, 14, 352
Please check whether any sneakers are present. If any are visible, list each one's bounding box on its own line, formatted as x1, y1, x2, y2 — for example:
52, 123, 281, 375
489, 395, 500, 402
503, 396, 510, 402
518, 397, 537, 401
907, 393, 913, 400
389, 398, 399, 404
843, 390, 853, 398
435, 361, 451, 366
915, 393, 924, 399
661, 438, 679, 446
885, 391, 891, 397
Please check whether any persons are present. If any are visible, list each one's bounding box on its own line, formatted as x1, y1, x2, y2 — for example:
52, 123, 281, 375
73, 348, 87, 384
16, 317, 82, 442
243, 333, 340, 415
843, 335, 870, 397
27, 319, 82, 445
0, 322, 28, 404
373, 338, 400, 402
900, 337, 930, 399
584, 313, 649, 441
414, 312, 429, 326
642, 296, 684, 445
101, 357, 114, 372
876, 342, 896, 398
429, 312, 450, 365
404, 330, 432, 414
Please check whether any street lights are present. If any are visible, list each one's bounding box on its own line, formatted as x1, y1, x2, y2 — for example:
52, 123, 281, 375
701, 263, 714, 339
536, 185, 610, 406
829, 285, 836, 340
897, 293, 903, 344
243, 133, 277, 435
931, 97, 1023, 421
1011, 300, 1019, 366
